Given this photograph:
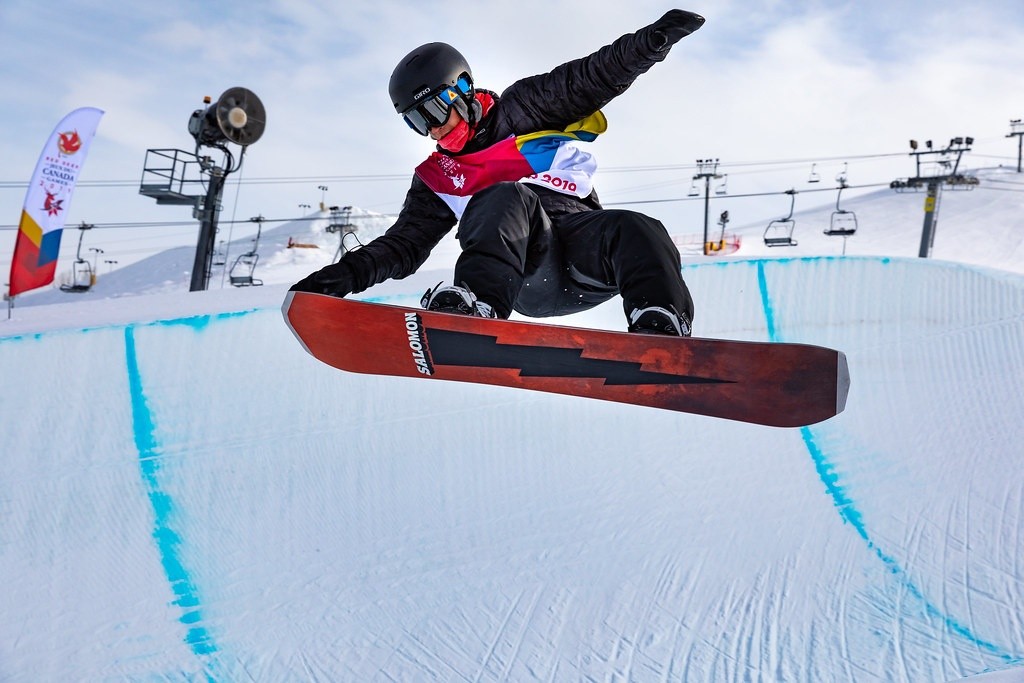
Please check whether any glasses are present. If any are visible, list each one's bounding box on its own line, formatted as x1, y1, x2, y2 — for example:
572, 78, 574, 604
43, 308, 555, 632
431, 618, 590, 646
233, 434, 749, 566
401, 79, 469, 137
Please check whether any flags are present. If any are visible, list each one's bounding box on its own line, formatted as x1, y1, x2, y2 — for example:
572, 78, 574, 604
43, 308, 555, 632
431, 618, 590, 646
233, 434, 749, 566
8, 105, 107, 299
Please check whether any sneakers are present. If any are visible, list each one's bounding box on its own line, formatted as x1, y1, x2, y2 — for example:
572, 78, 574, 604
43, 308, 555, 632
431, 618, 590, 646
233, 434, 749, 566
425, 284, 497, 318
629, 306, 685, 337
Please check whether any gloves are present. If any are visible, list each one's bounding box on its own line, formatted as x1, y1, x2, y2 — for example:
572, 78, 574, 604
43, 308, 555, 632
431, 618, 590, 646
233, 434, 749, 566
649, 9, 705, 51
288, 264, 351, 298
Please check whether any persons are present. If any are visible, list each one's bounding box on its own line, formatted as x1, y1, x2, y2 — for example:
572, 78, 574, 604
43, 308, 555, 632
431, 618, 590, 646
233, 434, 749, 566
288, 8, 705, 338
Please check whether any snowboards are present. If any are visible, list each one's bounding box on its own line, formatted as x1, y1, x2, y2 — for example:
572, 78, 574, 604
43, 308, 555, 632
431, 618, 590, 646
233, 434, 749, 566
279, 290, 853, 429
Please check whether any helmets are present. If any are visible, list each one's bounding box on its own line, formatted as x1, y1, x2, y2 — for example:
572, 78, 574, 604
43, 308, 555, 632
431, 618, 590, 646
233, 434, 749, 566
388, 42, 475, 137
